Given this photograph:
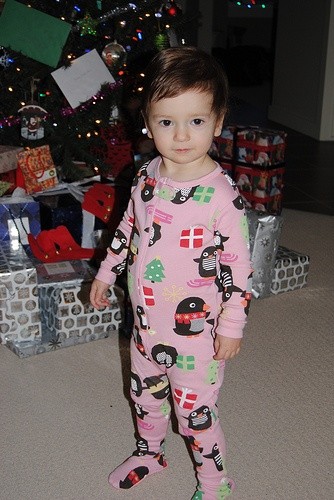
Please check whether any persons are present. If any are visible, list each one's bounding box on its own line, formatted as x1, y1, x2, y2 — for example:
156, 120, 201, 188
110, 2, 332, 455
88, 46, 254, 500
